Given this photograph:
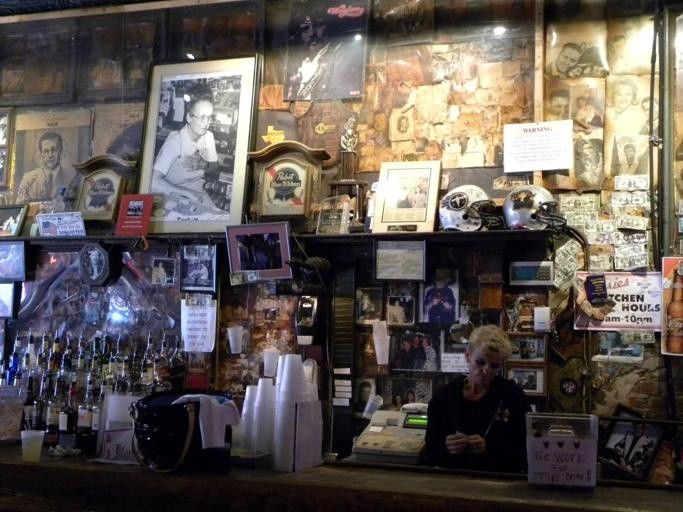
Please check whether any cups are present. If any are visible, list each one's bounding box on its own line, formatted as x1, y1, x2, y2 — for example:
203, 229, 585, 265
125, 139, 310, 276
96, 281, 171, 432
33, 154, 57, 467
225, 326, 243, 356
363, 394, 384, 419
371, 319, 390, 367
276, 353, 321, 476
240, 379, 276, 456
19, 430, 45, 462
262, 348, 279, 376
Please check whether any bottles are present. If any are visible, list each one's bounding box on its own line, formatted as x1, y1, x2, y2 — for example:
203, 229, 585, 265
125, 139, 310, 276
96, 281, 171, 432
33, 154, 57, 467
517, 295, 537, 331
45, 186, 68, 211
0, 328, 185, 454
667, 270, 683, 355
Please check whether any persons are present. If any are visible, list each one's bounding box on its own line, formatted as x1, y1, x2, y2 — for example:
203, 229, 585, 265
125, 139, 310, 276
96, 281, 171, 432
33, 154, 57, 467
153, 83, 221, 207
356, 272, 540, 411
616, 422, 650, 470
288, 2, 361, 98
183, 261, 209, 286
88, 249, 103, 278
545, 43, 656, 184
238, 236, 283, 270
155, 262, 167, 283
372, 111, 388, 146
17, 132, 75, 212
425, 325, 535, 481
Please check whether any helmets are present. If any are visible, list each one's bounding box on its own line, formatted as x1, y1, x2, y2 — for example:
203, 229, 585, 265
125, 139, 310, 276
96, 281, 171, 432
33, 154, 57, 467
500, 183, 568, 237
436, 181, 506, 234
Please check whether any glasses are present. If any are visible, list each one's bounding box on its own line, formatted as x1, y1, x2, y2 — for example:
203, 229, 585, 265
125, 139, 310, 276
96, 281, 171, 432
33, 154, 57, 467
198, 112, 217, 124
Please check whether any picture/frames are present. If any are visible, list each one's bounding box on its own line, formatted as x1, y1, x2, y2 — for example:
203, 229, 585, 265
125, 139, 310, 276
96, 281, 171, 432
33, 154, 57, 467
504, 332, 548, 396
225, 222, 293, 282
597, 402, 668, 482
353, 237, 459, 411
0, 203, 28, 320
138, 53, 262, 233
77, 243, 110, 287
371, 162, 441, 232
178, 241, 218, 292
6, 106, 96, 209
1, 2, 266, 112
